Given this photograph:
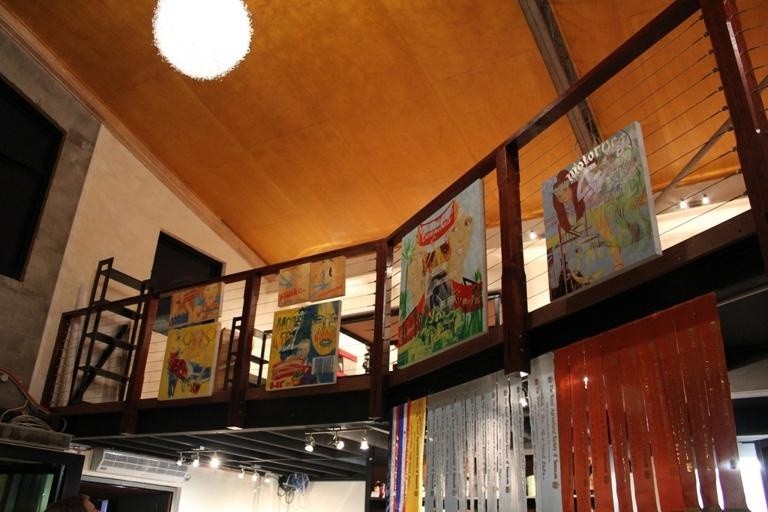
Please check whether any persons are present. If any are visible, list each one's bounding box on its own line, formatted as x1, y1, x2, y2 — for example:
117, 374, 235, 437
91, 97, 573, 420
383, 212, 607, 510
443, 207, 472, 279
552, 170, 623, 282
43, 494, 99, 512
271, 302, 337, 384
186, 284, 219, 321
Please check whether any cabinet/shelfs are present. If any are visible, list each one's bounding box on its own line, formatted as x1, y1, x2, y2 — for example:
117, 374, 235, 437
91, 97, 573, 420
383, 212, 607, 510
67, 256, 159, 408
221, 312, 275, 393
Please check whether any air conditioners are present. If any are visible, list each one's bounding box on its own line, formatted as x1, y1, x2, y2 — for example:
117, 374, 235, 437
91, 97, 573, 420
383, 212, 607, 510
88, 448, 190, 489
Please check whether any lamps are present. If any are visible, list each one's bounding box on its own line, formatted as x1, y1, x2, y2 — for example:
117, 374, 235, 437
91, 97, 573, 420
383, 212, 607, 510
303, 426, 372, 453
